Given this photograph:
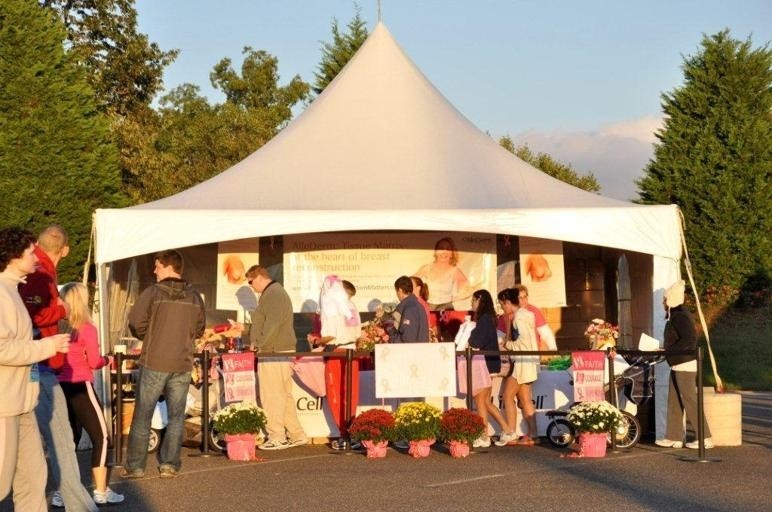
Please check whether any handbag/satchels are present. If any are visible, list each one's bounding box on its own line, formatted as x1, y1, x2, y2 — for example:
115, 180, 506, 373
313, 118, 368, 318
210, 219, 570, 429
456, 350, 491, 397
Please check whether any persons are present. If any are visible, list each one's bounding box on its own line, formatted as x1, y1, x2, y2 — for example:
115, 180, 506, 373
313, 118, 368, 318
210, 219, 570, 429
382, 275, 430, 403
458, 284, 562, 449
411, 236, 473, 312
0, 223, 126, 512
119, 249, 207, 478
651, 278, 716, 450
244, 264, 309, 451
409, 276, 431, 329
314, 273, 363, 451
306, 280, 356, 351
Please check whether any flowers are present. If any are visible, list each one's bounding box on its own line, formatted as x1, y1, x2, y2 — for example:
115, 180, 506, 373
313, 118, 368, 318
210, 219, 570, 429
566, 401, 627, 434
584, 319, 619, 350
348, 409, 397, 443
212, 402, 267, 433
441, 407, 485, 441
391, 402, 442, 439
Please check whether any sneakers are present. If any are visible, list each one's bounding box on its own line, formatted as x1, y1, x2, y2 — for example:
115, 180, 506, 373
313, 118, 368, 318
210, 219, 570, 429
472, 437, 491, 448
494, 434, 516, 446
686, 438, 714, 450
119, 468, 143, 479
160, 465, 177, 477
655, 439, 683, 449
287, 437, 308, 447
258, 440, 287, 450
94, 487, 123, 505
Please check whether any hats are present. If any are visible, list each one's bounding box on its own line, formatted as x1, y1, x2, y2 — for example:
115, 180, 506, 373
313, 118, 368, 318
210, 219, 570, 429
663, 280, 686, 309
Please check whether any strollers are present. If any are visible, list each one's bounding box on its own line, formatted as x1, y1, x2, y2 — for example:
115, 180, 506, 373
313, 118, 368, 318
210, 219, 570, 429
147, 348, 262, 456
545, 349, 667, 452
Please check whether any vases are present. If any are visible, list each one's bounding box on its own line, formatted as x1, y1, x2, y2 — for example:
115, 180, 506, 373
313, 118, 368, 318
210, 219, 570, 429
598, 336, 616, 349
408, 439, 436, 457
361, 440, 388, 457
578, 430, 607, 457
447, 439, 469, 457
223, 433, 257, 460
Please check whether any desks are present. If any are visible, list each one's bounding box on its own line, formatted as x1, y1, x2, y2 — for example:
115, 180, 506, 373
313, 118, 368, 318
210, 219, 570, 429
185, 349, 614, 444
